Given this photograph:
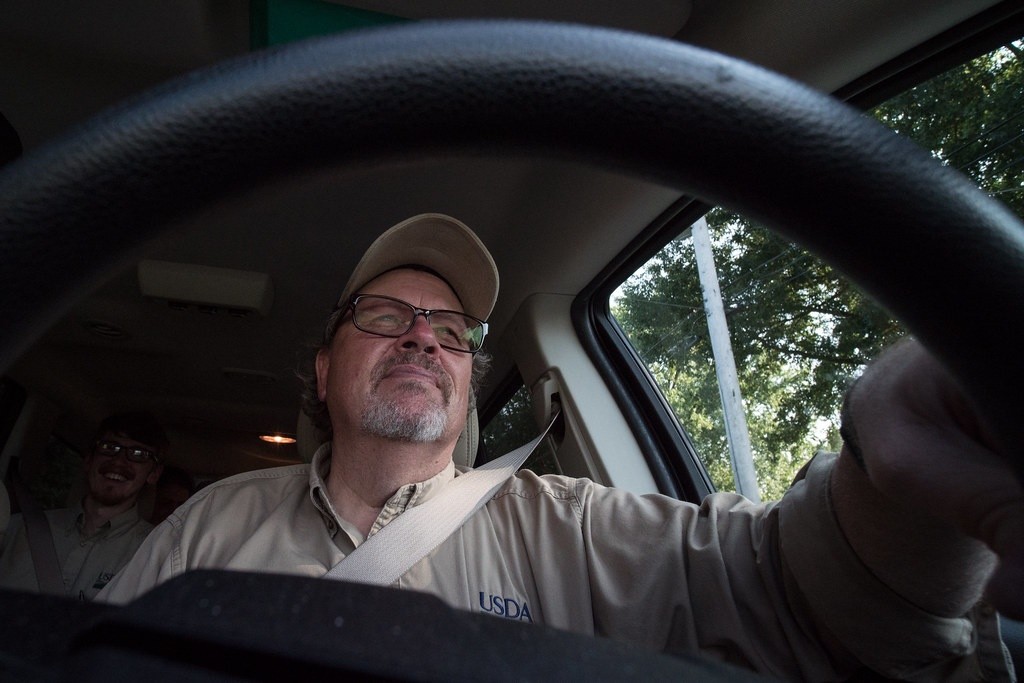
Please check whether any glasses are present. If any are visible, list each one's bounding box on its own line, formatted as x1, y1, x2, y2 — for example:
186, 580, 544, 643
328, 294, 488, 353
95, 439, 152, 463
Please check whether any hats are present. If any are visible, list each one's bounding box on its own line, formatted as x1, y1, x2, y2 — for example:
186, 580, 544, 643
338, 212, 500, 330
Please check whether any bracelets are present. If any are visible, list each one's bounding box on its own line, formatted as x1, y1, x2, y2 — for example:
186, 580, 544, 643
839, 375, 868, 473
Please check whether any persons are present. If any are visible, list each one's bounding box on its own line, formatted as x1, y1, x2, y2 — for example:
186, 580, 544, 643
92, 213, 1023, 683
0, 393, 213, 603
